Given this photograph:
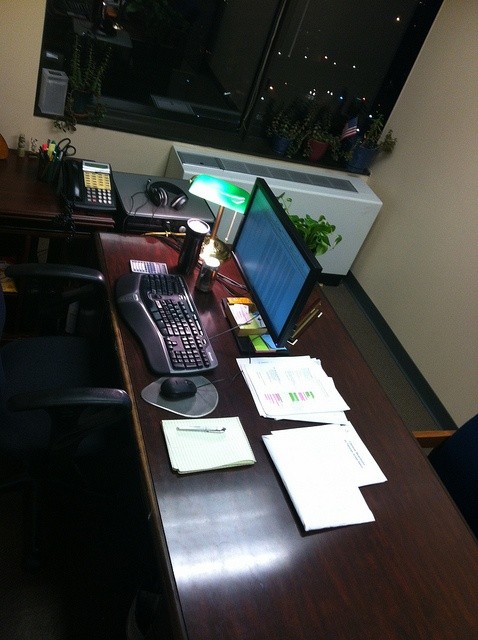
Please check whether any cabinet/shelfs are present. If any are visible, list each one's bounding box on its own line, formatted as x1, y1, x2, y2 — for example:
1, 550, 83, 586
0, 148, 116, 340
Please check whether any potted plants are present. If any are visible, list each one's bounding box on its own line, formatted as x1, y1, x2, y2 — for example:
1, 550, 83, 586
273, 108, 314, 159
345, 108, 390, 174
304, 115, 344, 164
66, 28, 111, 130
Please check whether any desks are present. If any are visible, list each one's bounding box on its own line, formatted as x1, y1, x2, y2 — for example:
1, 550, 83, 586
95, 228, 478, 640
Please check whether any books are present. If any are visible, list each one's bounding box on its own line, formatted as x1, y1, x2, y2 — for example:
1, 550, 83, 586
161, 415, 257, 477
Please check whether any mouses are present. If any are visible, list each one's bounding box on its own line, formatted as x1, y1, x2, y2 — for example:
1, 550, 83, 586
160, 377, 197, 400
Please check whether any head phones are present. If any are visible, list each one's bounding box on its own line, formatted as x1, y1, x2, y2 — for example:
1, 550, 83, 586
145, 177, 189, 210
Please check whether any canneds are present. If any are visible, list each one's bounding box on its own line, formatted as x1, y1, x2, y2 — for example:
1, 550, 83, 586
195, 256, 220, 293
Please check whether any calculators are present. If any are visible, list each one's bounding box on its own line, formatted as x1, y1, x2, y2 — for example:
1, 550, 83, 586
130, 259, 170, 276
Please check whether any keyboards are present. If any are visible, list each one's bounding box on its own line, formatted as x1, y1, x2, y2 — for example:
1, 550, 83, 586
114, 273, 220, 377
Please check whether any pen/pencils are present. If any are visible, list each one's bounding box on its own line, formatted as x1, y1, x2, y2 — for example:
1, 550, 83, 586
47, 138, 51, 152
176, 427, 226, 435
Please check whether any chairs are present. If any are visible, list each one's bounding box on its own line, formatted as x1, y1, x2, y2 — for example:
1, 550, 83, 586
429, 415, 475, 538
0, 262, 134, 571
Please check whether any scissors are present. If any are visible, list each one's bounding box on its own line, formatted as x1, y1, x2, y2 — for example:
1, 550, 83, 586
49, 138, 76, 166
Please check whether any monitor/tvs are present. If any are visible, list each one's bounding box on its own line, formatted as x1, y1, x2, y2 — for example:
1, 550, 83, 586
229, 178, 323, 346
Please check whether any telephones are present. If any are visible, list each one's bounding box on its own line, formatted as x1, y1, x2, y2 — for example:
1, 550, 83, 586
57, 159, 117, 228
